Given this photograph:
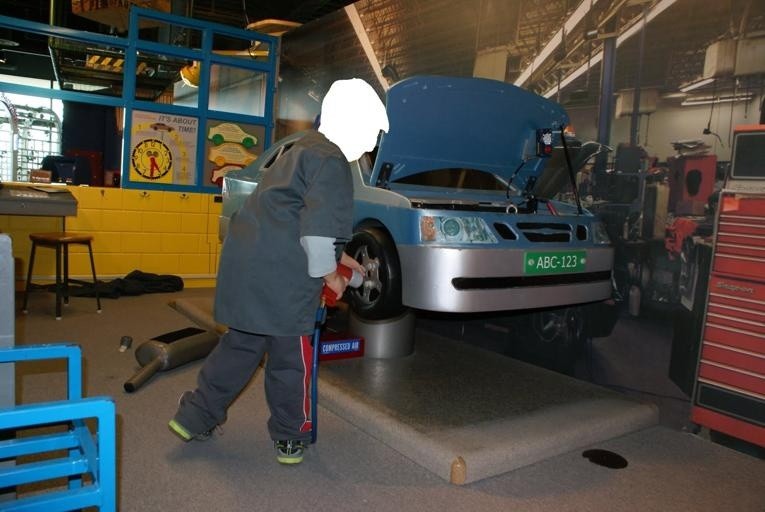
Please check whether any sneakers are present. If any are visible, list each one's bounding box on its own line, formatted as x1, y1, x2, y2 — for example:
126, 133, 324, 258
166, 411, 215, 444
272, 435, 307, 464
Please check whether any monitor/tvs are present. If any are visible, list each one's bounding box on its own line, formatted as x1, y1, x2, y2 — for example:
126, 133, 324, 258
727, 129, 765, 179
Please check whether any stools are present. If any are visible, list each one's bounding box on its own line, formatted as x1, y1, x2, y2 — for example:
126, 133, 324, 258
21, 230, 103, 321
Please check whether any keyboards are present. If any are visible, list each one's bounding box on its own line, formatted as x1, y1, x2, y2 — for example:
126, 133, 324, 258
722, 180, 765, 194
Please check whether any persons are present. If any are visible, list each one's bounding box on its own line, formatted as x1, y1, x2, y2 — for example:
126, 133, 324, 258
168, 76, 391, 467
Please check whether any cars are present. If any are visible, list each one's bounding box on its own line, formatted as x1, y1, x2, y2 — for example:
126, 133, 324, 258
219, 75, 616, 320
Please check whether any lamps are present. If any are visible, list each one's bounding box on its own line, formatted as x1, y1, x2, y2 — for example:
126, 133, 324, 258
550, 0, 599, 59
